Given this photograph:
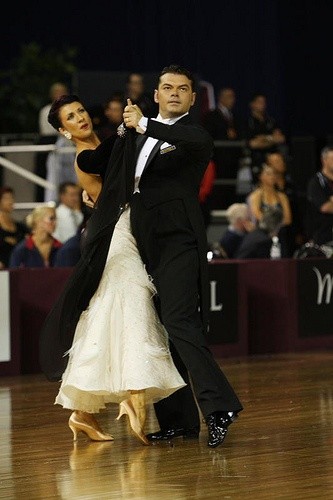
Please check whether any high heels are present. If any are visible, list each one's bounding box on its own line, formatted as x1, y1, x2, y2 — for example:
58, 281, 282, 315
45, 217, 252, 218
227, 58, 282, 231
115, 399, 151, 445
68, 412, 115, 442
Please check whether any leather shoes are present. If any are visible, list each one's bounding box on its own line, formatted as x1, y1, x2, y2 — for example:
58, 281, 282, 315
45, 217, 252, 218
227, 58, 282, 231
205, 409, 240, 449
145, 425, 199, 441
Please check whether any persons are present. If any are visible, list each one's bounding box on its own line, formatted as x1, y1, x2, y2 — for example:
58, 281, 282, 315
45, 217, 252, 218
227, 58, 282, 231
38, 83, 75, 137
261, 154, 301, 218
276, 224, 308, 258
119, 71, 155, 119
54, 183, 84, 243
220, 202, 255, 260
204, 87, 237, 140
81, 64, 245, 447
245, 163, 292, 259
9, 206, 65, 269
0, 188, 32, 269
237, 96, 287, 153
247, 227, 273, 260
306, 145, 333, 259
47, 95, 188, 445
101, 96, 126, 127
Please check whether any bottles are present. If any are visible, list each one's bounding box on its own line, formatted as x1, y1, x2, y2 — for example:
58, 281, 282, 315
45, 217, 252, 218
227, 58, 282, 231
270, 236, 281, 259
